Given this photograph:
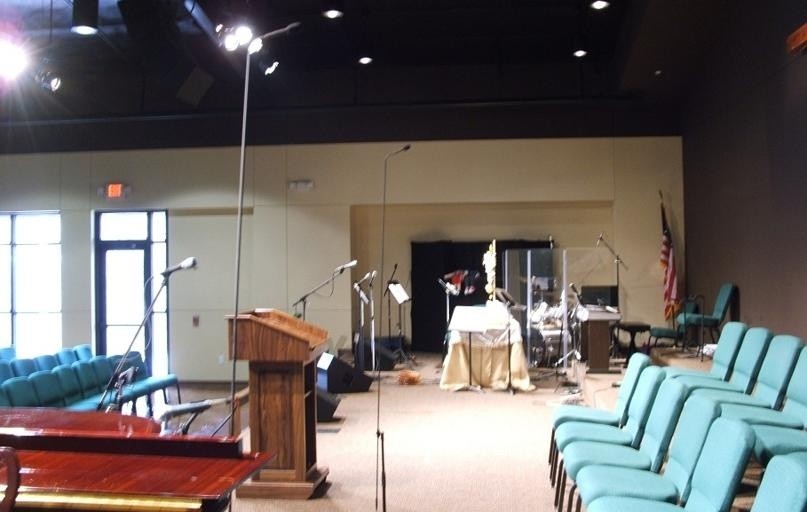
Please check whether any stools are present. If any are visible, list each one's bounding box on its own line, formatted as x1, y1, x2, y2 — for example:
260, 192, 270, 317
618, 320, 651, 367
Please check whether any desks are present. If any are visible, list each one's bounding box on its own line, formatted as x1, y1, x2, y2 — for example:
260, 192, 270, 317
576, 305, 621, 374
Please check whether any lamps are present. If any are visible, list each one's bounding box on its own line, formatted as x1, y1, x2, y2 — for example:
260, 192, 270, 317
71, 1, 100, 35
288, 178, 317, 193
215, 24, 239, 52
224, 22, 253, 46
98, 182, 134, 203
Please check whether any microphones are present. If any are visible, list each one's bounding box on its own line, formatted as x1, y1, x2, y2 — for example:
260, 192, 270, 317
437, 279, 449, 290
568, 282, 577, 295
161, 256, 197, 279
596, 231, 603, 246
550, 234, 556, 253
370, 271, 376, 288
333, 260, 357, 273
357, 273, 368, 285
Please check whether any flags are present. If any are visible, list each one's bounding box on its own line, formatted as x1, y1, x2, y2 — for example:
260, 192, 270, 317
659, 202, 682, 322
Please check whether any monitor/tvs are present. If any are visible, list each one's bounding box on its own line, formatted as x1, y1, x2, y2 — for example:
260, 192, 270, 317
580, 285, 618, 306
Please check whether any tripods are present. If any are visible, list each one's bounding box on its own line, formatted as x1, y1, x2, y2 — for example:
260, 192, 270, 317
389, 292, 418, 371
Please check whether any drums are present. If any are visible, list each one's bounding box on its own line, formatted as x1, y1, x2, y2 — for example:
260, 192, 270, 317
524, 325, 574, 367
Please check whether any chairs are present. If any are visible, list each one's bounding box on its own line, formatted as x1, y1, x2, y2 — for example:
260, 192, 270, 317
548, 351, 652, 465
647, 303, 698, 347
665, 321, 748, 381
722, 345, 807, 426
550, 364, 667, 488
112, 350, 181, 415
554, 377, 688, 512
749, 423, 807, 455
567, 390, 720, 512
751, 455, 807, 512
677, 325, 772, 390
678, 281, 735, 354
1, 354, 112, 410
694, 333, 802, 406
1, 347, 94, 379
588, 416, 755, 512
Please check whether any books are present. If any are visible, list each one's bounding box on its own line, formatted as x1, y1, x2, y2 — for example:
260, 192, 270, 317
389, 283, 410, 304
